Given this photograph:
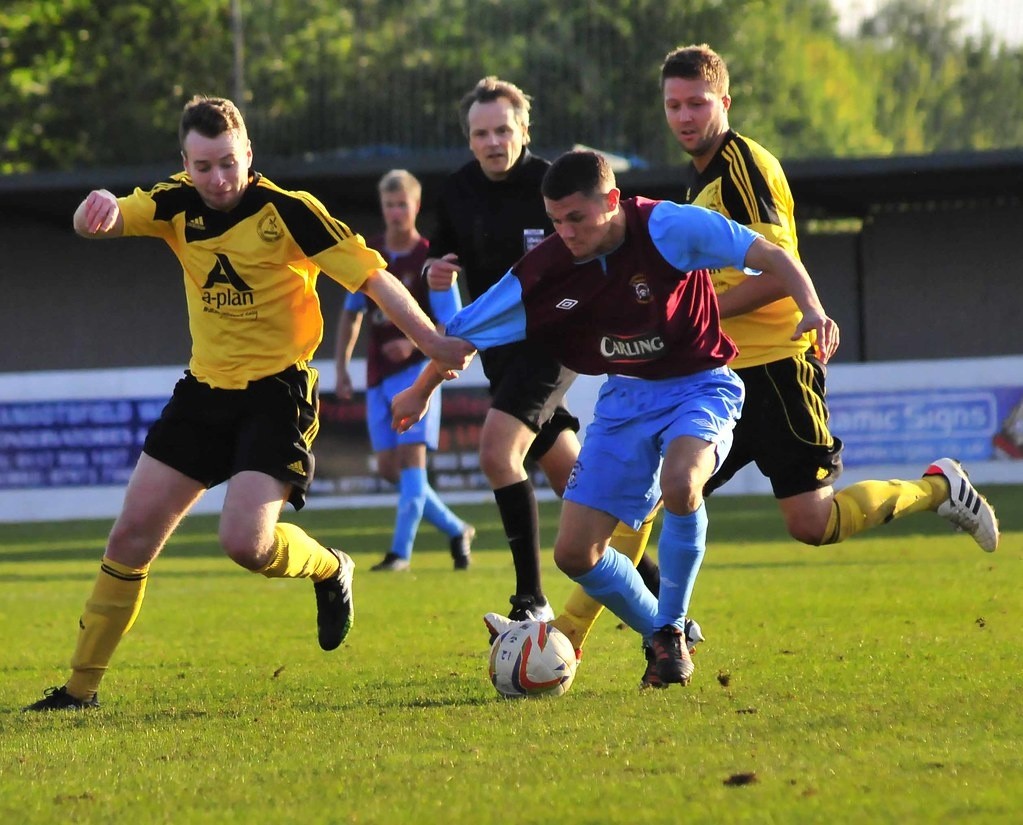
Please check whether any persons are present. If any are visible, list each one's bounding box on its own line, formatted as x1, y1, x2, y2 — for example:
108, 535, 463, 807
391, 152, 839, 689
421, 81, 662, 647
336, 170, 475, 572
485, 44, 999, 662
25, 96, 477, 709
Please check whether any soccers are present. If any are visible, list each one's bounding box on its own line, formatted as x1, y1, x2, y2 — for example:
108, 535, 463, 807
487, 620, 580, 699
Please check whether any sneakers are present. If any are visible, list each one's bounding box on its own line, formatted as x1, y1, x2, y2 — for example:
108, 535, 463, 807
451, 525, 475, 570
483, 613, 582, 669
922, 458, 999, 553
640, 619, 705, 690
312, 547, 354, 651
372, 553, 410, 572
490, 594, 554, 646
22, 683, 99, 714
646, 622, 695, 685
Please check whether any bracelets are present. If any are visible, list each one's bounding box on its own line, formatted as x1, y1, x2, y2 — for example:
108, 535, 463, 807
421, 264, 431, 288
